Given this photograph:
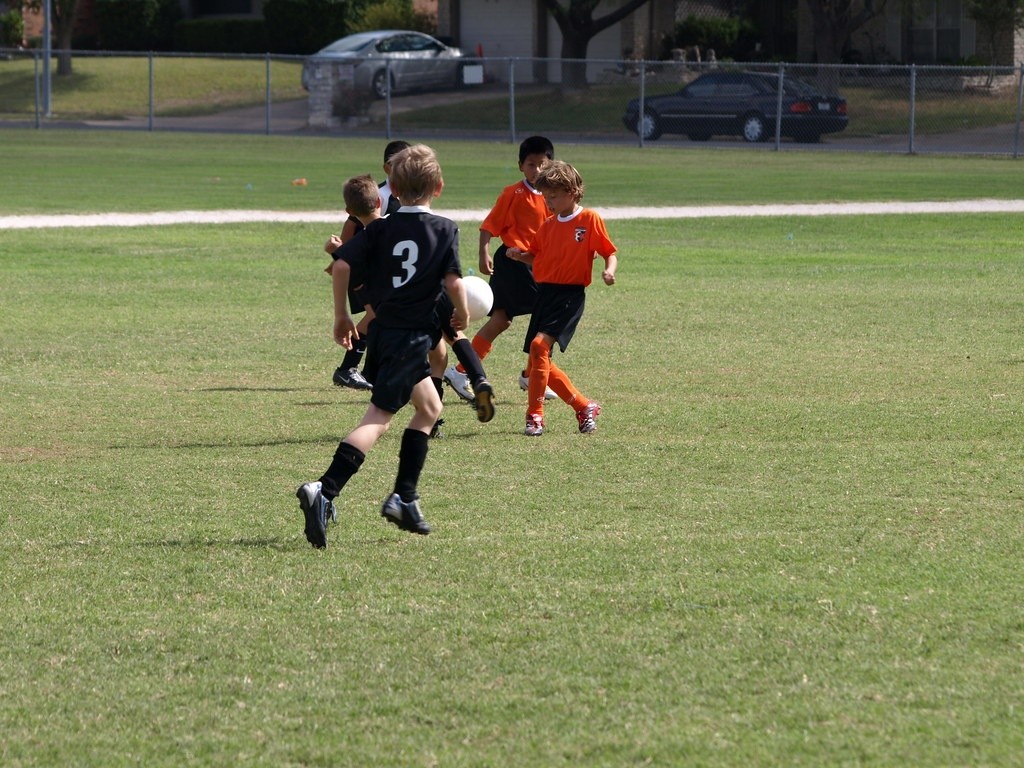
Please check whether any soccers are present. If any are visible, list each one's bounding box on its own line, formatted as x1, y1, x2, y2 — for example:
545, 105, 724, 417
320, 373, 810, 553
461, 275, 494, 322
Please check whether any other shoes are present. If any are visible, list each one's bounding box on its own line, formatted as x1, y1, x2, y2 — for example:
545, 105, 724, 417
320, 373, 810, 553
431, 418, 443, 438
471, 376, 501, 421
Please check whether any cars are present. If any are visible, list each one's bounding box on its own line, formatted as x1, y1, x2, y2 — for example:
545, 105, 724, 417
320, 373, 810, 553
301, 30, 482, 99
623, 70, 850, 143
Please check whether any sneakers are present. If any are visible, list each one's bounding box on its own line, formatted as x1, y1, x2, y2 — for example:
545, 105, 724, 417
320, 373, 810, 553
443, 364, 475, 403
333, 366, 374, 391
296, 480, 337, 550
382, 492, 431, 536
523, 414, 545, 437
576, 399, 602, 434
518, 369, 559, 400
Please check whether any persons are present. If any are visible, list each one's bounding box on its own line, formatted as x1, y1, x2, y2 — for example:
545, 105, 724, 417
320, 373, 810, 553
323, 139, 495, 438
296, 142, 469, 550
506, 159, 617, 436
622, 47, 716, 75
443, 135, 561, 403
